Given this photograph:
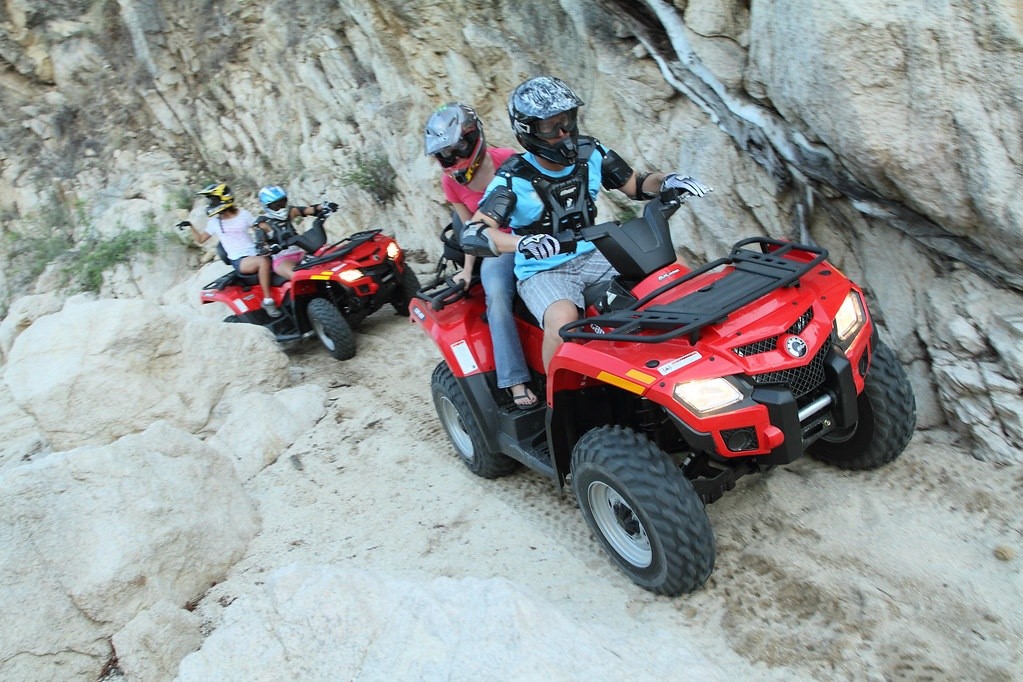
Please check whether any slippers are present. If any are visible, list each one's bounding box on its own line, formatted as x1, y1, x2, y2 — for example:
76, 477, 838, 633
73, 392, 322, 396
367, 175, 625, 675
507, 383, 538, 410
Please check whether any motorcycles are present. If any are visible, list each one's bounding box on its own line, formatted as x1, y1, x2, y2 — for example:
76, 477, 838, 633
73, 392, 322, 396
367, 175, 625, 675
407, 180, 917, 598
200, 205, 422, 361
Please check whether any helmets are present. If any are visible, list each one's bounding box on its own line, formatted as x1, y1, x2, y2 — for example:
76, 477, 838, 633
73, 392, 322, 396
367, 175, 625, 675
424, 101, 485, 184
258, 185, 288, 220
195, 183, 236, 217
506, 76, 585, 166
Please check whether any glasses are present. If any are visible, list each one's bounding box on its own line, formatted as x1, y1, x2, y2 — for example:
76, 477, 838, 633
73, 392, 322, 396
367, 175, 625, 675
529, 109, 576, 138
436, 130, 480, 168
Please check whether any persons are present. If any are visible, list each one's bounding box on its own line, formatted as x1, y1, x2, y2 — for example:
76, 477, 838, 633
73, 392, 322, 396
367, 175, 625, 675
423, 102, 540, 409
461, 75, 707, 375
249, 185, 338, 280
175, 184, 281, 317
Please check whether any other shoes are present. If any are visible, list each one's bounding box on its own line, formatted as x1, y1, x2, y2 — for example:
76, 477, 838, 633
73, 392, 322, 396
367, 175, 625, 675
261, 300, 281, 319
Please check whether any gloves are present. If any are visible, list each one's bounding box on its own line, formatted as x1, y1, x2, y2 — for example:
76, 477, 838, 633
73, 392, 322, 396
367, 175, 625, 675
660, 172, 707, 198
518, 234, 561, 260
175, 221, 191, 231
322, 201, 339, 212
270, 243, 282, 254
